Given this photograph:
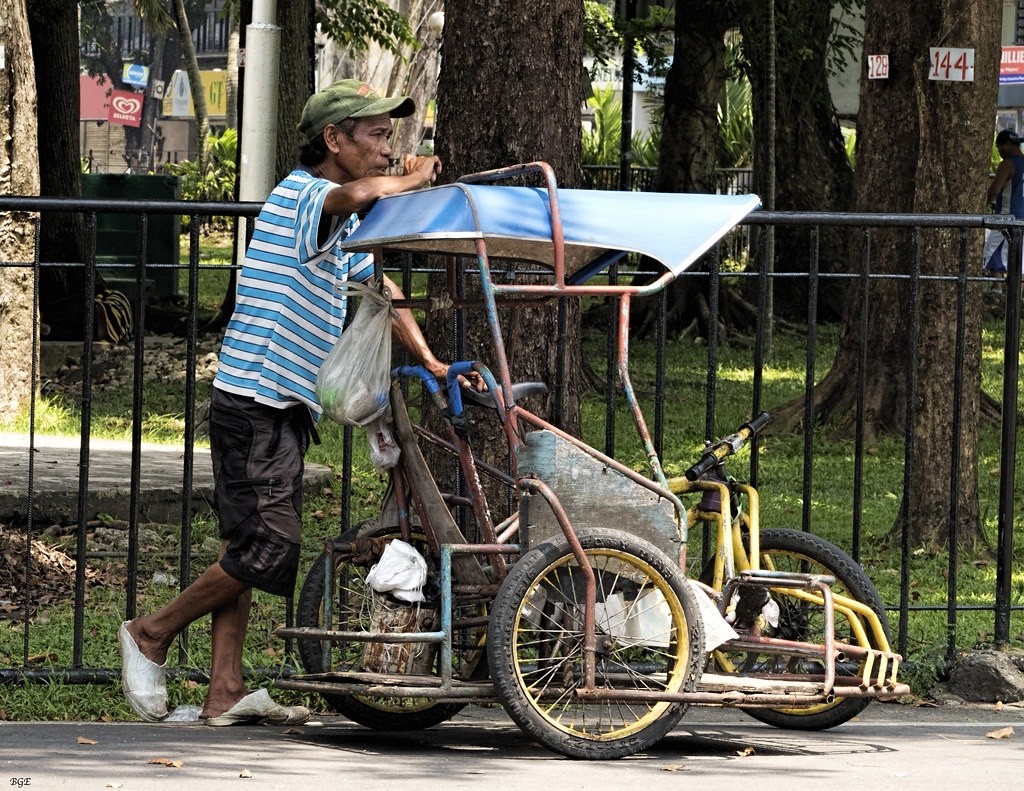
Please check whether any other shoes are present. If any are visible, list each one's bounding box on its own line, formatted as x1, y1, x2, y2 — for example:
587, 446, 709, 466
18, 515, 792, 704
983, 287, 1002, 294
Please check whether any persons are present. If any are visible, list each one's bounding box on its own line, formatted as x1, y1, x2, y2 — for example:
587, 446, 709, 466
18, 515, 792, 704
114, 78, 490, 726
982, 128, 1023, 273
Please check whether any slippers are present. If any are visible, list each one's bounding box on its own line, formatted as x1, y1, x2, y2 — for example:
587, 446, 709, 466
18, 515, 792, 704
116, 620, 169, 723
204, 688, 312, 727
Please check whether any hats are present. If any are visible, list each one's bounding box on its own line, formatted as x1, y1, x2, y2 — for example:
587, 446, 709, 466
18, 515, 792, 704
296, 78, 417, 149
996, 129, 1024, 145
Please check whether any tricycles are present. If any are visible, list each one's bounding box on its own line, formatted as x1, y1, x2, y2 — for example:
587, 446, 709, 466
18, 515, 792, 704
272, 161, 911, 762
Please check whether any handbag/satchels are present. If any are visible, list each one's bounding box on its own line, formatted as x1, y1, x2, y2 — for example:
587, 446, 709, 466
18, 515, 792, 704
315, 281, 402, 427
